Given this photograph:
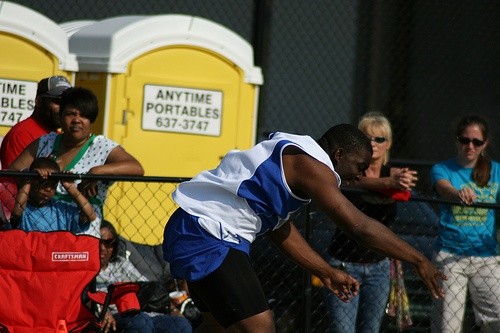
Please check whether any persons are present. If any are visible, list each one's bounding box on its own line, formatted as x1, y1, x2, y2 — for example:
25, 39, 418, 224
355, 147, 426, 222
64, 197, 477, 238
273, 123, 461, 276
8, 86, 144, 217
162, 123, 447, 333
96, 219, 192, 333
11, 157, 96, 234
0, 76, 73, 232
430, 116, 500, 333
327, 111, 418, 333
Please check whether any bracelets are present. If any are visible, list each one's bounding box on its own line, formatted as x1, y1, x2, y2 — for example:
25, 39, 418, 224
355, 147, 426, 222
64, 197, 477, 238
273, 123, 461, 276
171, 307, 179, 312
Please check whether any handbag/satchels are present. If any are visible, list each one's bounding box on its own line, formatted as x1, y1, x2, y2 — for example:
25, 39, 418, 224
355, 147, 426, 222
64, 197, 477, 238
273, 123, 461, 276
137, 281, 170, 315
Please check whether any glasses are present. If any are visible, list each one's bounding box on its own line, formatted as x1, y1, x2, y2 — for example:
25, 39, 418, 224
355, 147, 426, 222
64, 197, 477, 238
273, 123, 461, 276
100, 238, 117, 248
458, 136, 484, 147
370, 137, 386, 143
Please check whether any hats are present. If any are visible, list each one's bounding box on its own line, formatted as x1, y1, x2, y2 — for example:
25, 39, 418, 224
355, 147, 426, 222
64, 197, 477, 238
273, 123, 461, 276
37, 75, 72, 98
